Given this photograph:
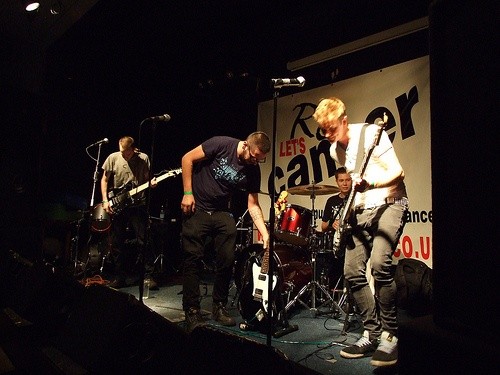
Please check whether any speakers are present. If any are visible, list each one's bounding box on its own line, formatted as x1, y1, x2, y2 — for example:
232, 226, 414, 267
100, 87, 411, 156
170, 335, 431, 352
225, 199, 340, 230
12, 255, 292, 375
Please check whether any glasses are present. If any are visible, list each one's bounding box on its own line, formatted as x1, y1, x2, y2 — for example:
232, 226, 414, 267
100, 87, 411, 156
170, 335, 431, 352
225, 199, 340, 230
248, 147, 267, 163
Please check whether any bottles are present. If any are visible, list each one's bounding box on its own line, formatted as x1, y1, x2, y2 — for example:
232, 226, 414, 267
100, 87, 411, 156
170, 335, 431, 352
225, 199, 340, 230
142, 277, 151, 300
160, 203, 164, 220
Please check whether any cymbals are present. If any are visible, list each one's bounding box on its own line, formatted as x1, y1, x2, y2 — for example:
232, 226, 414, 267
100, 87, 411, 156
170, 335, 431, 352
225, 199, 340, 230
287, 184, 340, 196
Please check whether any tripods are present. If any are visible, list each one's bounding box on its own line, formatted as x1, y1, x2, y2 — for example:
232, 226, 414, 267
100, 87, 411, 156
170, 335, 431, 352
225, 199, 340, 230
276, 192, 350, 323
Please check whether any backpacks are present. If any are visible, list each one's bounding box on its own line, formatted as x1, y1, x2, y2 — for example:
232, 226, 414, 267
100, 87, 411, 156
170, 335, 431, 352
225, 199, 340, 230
393, 258, 432, 307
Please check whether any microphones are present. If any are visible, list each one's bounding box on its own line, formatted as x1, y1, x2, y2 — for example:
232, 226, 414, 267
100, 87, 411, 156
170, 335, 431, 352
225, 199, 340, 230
90, 138, 109, 147
270, 76, 305, 87
148, 114, 171, 122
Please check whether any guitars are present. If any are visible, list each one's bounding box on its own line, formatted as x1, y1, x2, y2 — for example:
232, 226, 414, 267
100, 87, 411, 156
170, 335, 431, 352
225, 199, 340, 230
238, 190, 288, 324
86, 167, 183, 233
332, 110, 390, 255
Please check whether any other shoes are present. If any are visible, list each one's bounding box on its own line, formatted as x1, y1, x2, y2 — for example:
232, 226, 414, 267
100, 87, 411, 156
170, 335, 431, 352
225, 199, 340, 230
108, 275, 126, 287
143, 274, 159, 290
212, 308, 237, 326
184, 306, 209, 331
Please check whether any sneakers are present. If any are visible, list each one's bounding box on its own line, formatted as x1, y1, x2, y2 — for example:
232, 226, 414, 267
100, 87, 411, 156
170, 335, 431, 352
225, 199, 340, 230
369, 331, 398, 367
339, 329, 379, 359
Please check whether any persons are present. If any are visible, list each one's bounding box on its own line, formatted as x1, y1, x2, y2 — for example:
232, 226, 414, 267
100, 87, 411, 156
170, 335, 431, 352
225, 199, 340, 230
321, 168, 352, 232
312, 97, 408, 366
100, 136, 158, 289
182, 132, 271, 326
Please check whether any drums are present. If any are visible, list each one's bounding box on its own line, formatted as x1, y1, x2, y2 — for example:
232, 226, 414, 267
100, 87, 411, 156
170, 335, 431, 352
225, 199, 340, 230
273, 202, 314, 247
307, 226, 335, 254
65, 221, 115, 278
234, 243, 321, 314
257, 221, 272, 243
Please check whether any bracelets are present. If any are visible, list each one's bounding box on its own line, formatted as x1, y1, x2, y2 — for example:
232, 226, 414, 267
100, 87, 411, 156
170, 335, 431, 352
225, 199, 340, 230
184, 192, 193, 195
